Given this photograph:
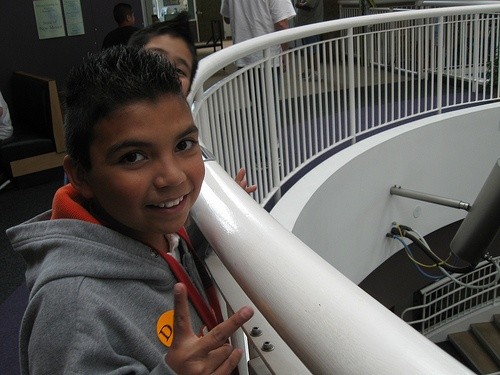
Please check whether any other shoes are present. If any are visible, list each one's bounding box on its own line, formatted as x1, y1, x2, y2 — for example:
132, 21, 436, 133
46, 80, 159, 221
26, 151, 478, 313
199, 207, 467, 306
302, 69, 325, 82
269, 139, 282, 151
252, 159, 283, 171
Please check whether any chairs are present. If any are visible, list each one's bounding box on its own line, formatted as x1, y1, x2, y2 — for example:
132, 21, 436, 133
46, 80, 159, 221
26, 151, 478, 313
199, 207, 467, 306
5, 68, 69, 178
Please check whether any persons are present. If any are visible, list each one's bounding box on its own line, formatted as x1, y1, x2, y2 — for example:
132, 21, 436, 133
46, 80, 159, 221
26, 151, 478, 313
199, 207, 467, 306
293, 0, 326, 82
6, 43, 255, 375
219, 0, 297, 174
0, 86, 13, 143
102, 0, 140, 50
126, 11, 257, 262
